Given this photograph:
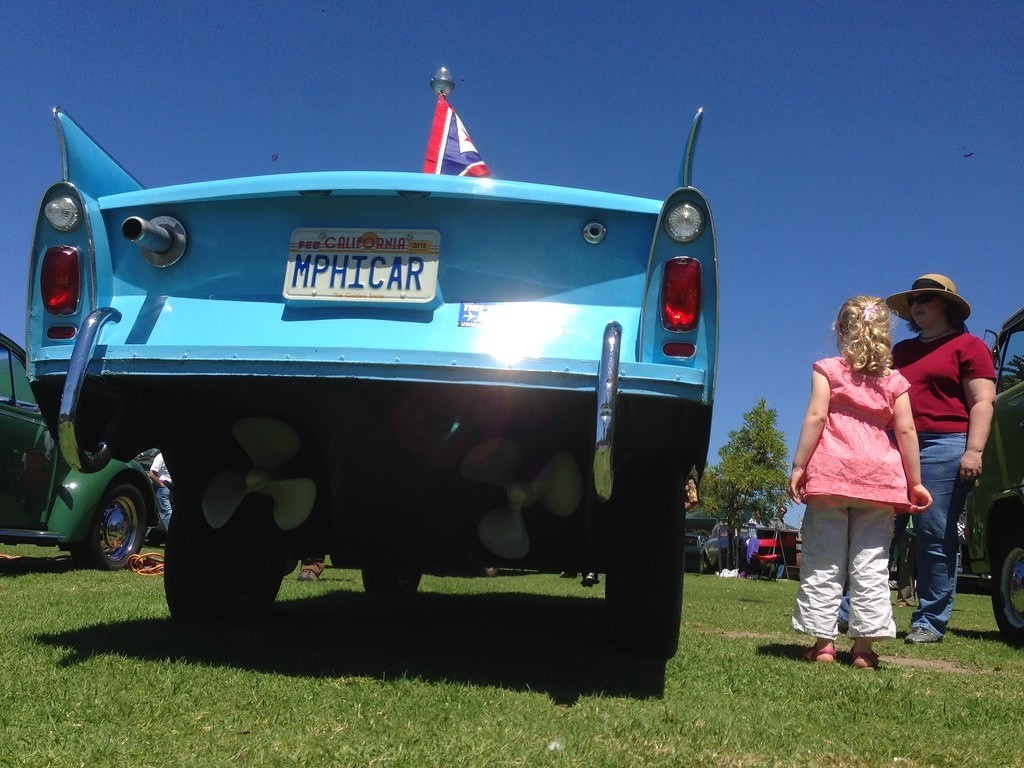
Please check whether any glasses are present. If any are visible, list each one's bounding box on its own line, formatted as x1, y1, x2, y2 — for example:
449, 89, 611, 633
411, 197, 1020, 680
908, 292, 932, 306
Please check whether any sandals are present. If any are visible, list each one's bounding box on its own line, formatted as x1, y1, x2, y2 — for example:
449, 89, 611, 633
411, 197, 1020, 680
802, 642, 838, 665
849, 646, 879, 669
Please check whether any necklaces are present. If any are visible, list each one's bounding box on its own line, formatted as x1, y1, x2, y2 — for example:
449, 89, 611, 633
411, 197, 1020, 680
919, 328, 955, 339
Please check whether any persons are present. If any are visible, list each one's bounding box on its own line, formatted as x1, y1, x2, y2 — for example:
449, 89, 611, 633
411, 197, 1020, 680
149, 451, 172, 532
788, 295, 932, 672
837, 274, 996, 644
298, 562, 324, 581
776, 507, 787, 522
897, 515, 919, 607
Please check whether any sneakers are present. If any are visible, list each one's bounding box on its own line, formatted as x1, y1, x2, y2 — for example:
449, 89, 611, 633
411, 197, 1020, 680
896, 601, 907, 608
903, 626, 941, 643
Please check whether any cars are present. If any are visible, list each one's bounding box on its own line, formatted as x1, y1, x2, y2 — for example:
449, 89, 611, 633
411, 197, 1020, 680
983, 303, 1024, 647
24, 66, 718, 659
0, 331, 159, 572
683, 507, 970, 596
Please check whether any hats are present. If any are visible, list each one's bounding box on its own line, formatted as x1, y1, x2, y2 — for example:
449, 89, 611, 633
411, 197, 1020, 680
885, 274, 970, 323
